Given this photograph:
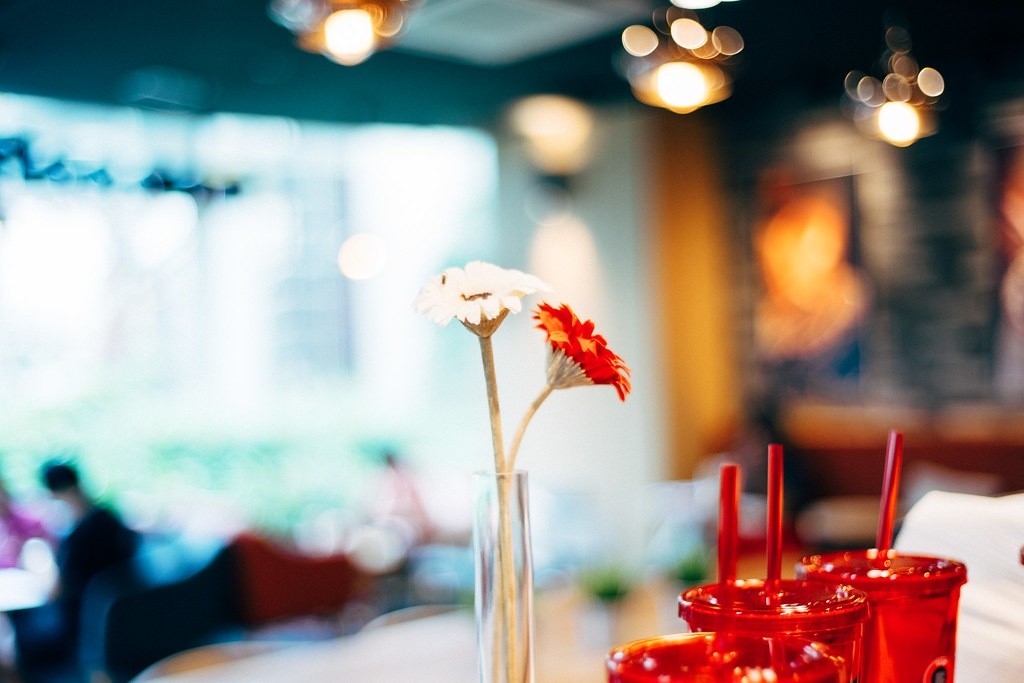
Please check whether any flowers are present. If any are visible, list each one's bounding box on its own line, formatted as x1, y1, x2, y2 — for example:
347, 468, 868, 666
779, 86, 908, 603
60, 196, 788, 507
414, 259, 630, 683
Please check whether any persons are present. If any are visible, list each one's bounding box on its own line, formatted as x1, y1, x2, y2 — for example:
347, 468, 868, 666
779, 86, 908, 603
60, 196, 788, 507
0, 485, 57, 568
16, 464, 137, 683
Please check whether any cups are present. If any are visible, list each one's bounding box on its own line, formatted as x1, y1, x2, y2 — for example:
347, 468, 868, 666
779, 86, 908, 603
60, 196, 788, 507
606, 548, 968, 683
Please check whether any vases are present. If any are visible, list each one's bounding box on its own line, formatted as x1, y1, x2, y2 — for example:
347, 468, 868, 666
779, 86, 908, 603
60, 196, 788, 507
464, 472, 536, 683
